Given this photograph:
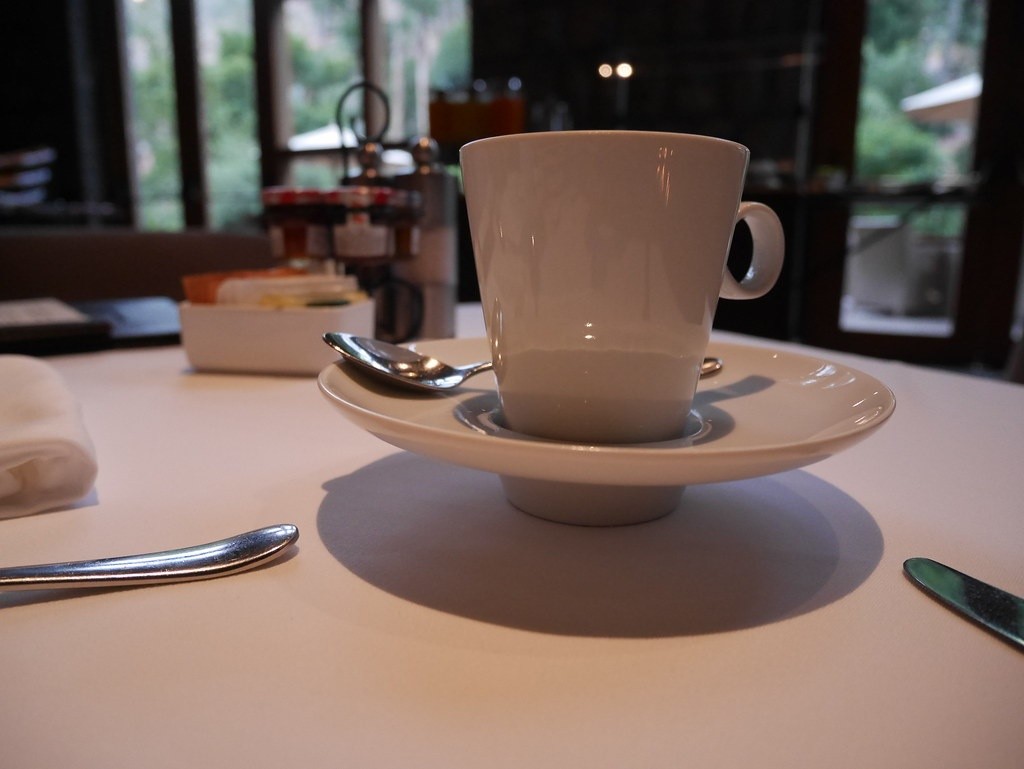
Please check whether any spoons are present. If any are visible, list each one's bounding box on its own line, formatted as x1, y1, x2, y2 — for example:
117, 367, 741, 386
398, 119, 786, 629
322, 331, 725, 395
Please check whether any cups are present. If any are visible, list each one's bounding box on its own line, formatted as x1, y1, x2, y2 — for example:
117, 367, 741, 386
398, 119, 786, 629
457, 130, 785, 440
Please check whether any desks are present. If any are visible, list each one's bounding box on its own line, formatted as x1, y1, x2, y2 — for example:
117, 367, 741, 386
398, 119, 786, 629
0, 304, 1023, 769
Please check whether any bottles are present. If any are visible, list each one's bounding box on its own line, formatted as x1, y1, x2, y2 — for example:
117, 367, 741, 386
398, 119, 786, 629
395, 140, 458, 344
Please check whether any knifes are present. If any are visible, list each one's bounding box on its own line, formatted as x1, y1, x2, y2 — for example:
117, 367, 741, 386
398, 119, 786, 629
904, 557, 1024, 649
